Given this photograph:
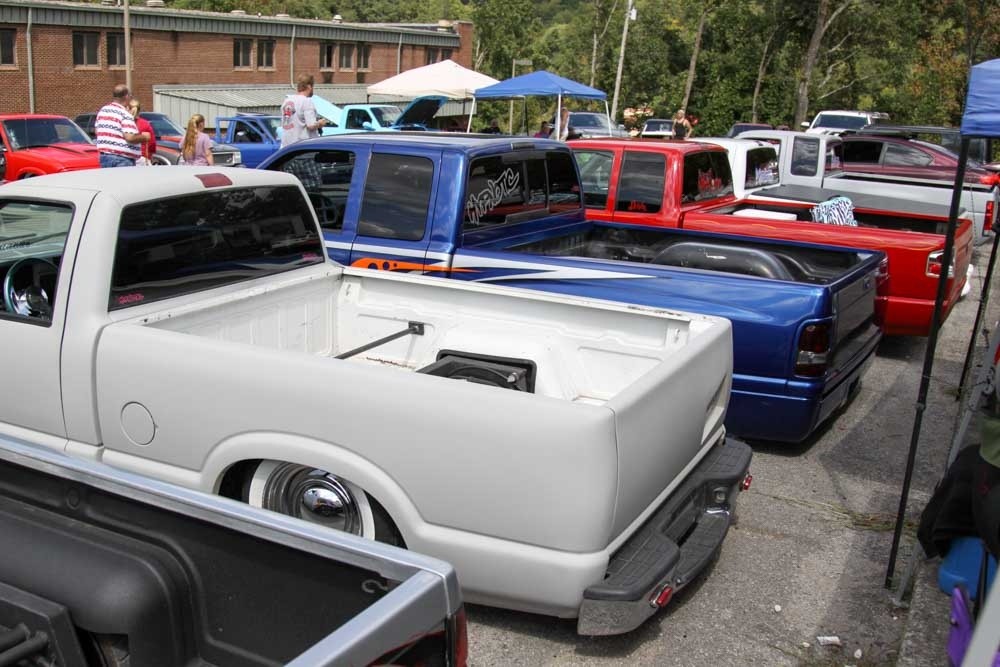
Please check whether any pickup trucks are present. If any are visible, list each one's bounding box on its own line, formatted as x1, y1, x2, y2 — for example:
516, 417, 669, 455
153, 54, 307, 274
0, 434, 469, 667
683, 130, 1000, 248
254, 129, 889, 444
566, 138, 975, 337
0, 165, 754, 636
209, 114, 283, 169
276, 94, 452, 143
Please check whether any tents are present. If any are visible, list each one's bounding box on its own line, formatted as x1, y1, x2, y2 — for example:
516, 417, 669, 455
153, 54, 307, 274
883, 55, 1000, 589
465, 71, 613, 141
366, 58, 530, 138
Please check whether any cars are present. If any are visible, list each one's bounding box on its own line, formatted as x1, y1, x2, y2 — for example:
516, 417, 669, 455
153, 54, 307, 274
68, 111, 250, 169
0, 113, 102, 185
549, 112, 631, 140
726, 123, 773, 139
833, 125, 1000, 185
640, 118, 675, 139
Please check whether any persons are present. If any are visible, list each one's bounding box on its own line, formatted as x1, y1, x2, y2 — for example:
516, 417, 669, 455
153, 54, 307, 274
94, 84, 157, 168
478, 119, 500, 134
672, 109, 692, 140
533, 96, 583, 142
178, 113, 214, 167
280, 72, 329, 149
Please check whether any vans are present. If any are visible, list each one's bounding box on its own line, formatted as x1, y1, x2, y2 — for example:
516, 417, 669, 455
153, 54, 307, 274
800, 109, 892, 137
